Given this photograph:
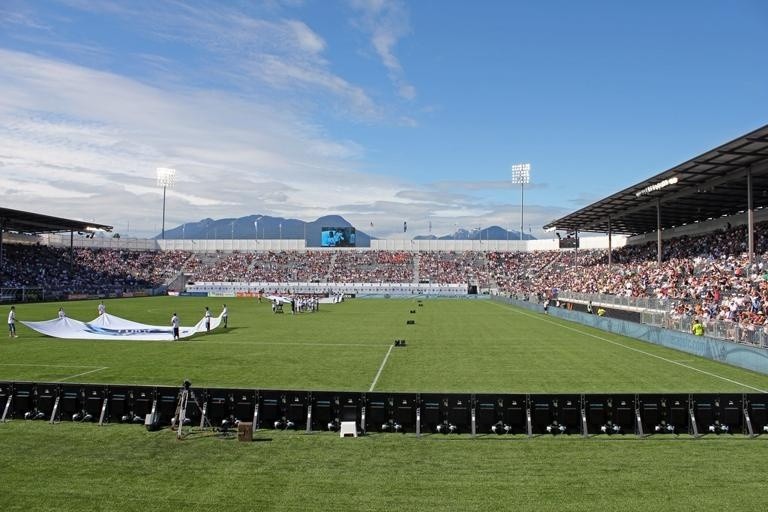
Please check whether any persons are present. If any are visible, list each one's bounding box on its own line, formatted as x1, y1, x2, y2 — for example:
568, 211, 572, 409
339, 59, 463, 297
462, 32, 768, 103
533, 220, 768, 351
204, 306, 211, 334
7, 306, 18, 338
221, 304, 229, 328
58, 307, 65, 318
97, 300, 105, 315
171, 312, 180, 341
418, 248, 560, 301
1, 231, 191, 301
258, 289, 290, 303
186, 248, 416, 286
269, 296, 319, 315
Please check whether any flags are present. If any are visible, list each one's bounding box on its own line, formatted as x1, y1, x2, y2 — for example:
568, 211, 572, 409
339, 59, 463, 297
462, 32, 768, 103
18, 310, 225, 341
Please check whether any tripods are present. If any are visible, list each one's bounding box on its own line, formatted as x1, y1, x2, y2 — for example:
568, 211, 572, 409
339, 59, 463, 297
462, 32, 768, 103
170, 389, 214, 440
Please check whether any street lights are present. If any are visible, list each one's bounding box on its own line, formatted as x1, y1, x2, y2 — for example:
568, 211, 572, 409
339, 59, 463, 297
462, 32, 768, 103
511, 162, 531, 240
156, 167, 176, 238
